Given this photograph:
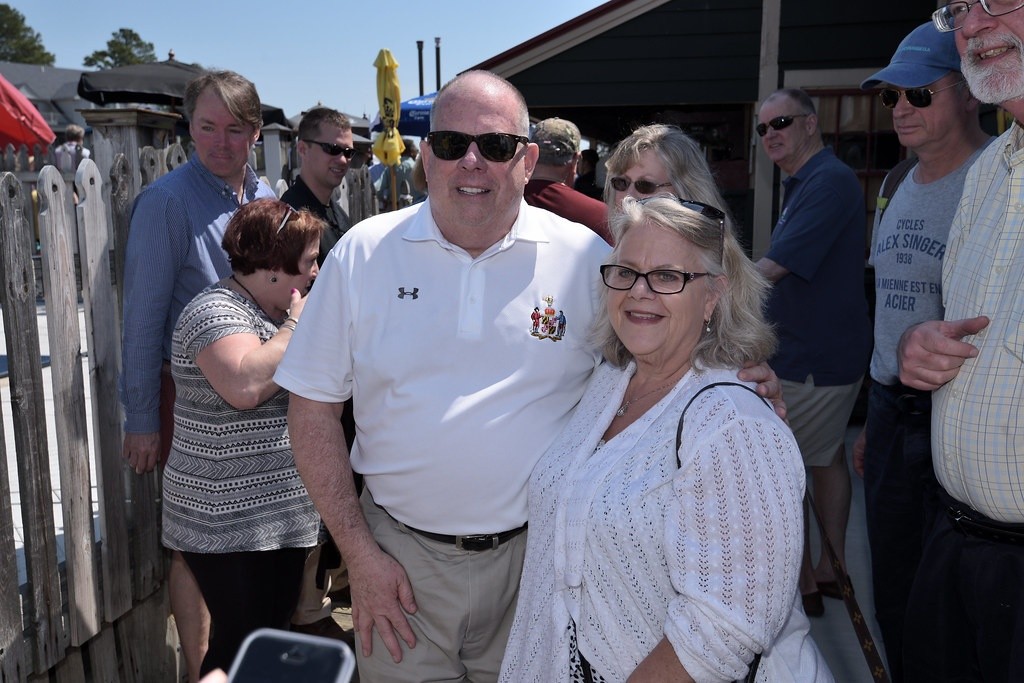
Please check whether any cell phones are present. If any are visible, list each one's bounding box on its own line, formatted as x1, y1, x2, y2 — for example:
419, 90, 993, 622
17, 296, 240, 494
225, 629, 356, 683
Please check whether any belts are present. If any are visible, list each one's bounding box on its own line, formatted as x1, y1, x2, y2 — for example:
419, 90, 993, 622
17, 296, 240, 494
386, 509, 529, 552
942, 488, 1024, 545
874, 381, 931, 415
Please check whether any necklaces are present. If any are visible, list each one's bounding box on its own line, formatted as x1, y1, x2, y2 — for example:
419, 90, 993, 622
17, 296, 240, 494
230, 275, 279, 329
617, 377, 681, 417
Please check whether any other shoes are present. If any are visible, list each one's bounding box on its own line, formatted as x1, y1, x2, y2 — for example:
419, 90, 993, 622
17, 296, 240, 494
802, 591, 824, 617
289, 618, 352, 644
817, 576, 854, 598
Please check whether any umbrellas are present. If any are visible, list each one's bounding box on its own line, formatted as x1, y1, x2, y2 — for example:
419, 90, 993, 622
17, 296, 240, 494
370, 93, 437, 136
373, 50, 407, 210
78, 50, 292, 144
287, 102, 370, 149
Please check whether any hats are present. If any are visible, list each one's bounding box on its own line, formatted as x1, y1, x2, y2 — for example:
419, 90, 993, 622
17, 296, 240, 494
530, 116, 581, 157
860, 17, 961, 90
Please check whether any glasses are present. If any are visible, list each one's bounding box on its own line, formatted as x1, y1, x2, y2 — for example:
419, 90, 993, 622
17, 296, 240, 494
601, 261, 708, 294
756, 113, 811, 136
637, 197, 726, 266
303, 138, 356, 160
427, 130, 530, 162
610, 177, 672, 194
932, 0, 1024, 32
880, 79, 966, 109
276, 205, 298, 236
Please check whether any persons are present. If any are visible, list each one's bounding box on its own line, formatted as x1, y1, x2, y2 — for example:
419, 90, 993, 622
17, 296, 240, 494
367, 138, 429, 214
278, 109, 365, 659
524, 118, 615, 248
752, 89, 872, 619
496, 192, 836, 683
199, 669, 229, 683
161, 197, 320, 683
121, 70, 277, 683
573, 149, 605, 203
897, 0, 1024, 683
853, 17, 998, 683
603, 123, 733, 243
28, 124, 90, 244
272, 70, 790, 683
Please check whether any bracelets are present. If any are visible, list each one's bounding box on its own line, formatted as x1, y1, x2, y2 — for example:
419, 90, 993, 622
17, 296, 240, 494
285, 318, 297, 324
281, 324, 295, 331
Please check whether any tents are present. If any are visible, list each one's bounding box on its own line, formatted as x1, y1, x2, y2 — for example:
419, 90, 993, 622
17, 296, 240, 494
0, 72, 56, 157
